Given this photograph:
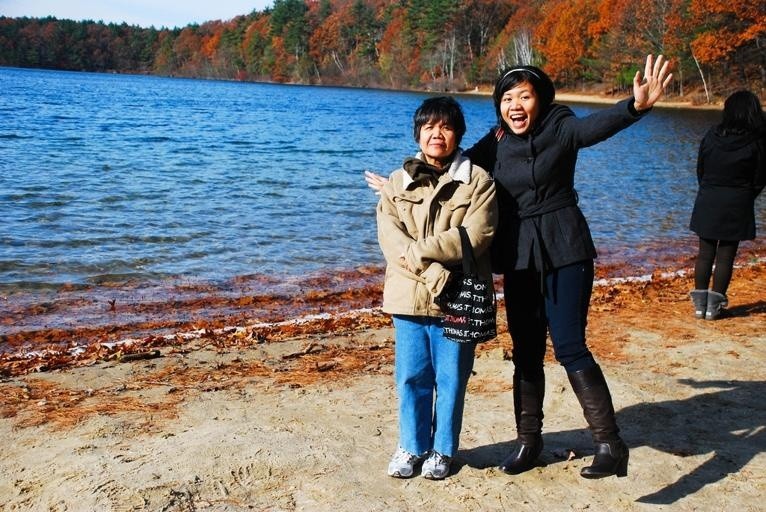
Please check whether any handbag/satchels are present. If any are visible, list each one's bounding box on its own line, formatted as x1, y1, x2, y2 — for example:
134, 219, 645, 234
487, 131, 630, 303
442, 268, 498, 343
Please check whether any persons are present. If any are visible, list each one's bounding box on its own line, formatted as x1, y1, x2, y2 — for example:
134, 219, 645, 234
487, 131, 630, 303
364, 53, 675, 480
376, 93, 499, 482
686, 89, 764, 322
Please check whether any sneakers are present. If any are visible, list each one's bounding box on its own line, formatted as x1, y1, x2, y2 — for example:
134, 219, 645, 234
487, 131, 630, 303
388, 447, 420, 478
421, 450, 452, 480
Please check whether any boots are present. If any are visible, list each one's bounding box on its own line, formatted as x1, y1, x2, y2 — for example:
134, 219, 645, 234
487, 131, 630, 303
567, 364, 629, 478
499, 374, 545, 475
690, 290, 707, 318
705, 291, 727, 320
